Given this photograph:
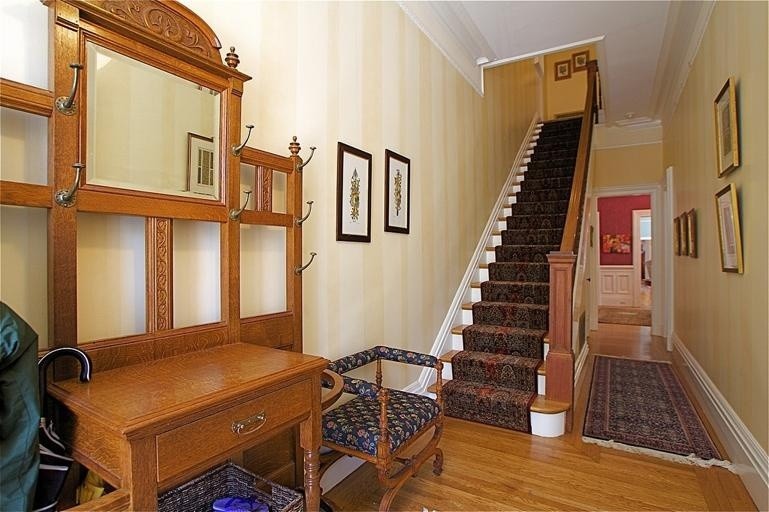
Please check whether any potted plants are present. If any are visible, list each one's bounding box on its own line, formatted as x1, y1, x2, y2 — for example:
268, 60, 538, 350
714, 182, 745, 276
671, 206, 698, 259
335, 140, 372, 243
186, 131, 214, 198
571, 50, 590, 73
554, 59, 571, 82
382, 148, 412, 235
712, 75, 741, 179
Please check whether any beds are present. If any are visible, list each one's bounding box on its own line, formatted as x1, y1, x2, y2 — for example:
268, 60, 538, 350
35, 344, 92, 511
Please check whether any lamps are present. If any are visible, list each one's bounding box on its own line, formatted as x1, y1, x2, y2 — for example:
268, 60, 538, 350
315, 344, 446, 511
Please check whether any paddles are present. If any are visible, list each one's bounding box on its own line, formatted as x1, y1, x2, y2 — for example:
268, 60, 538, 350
579, 352, 740, 474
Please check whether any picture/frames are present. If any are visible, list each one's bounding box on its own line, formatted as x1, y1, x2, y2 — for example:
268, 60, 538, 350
50, 341, 332, 512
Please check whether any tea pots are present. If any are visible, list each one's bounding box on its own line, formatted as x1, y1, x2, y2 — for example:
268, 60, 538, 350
79, 28, 228, 208
